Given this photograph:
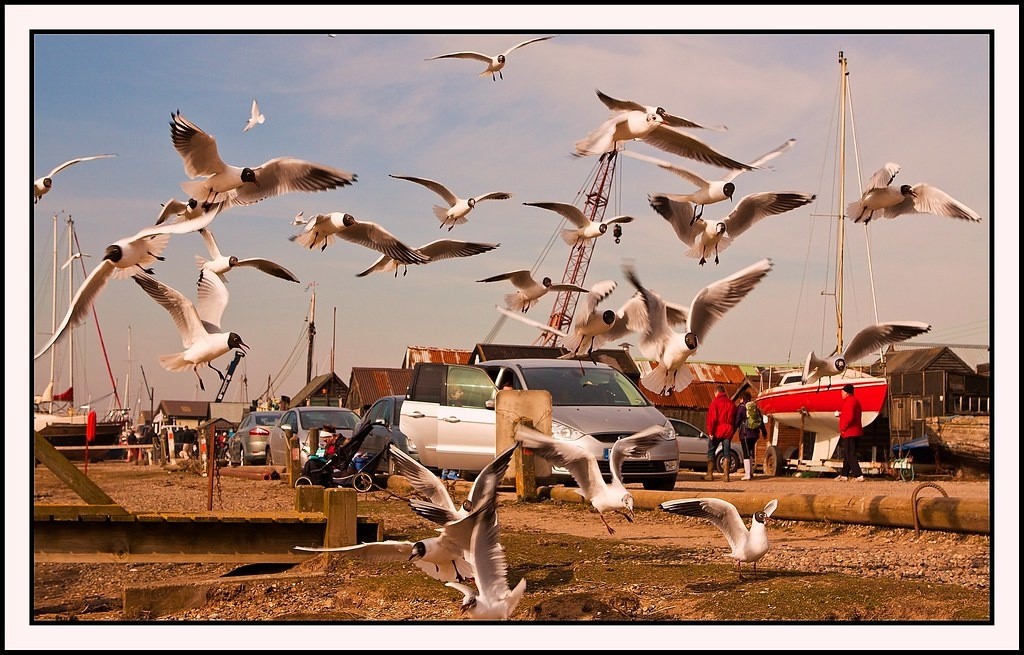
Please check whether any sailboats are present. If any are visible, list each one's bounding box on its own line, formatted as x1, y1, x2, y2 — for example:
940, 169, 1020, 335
755, 45, 892, 463
32, 208, 132, 461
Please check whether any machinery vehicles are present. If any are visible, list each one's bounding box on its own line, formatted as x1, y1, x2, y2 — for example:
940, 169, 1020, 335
482, 149, 626, 347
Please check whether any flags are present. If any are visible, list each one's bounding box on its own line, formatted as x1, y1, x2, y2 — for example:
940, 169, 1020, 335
52, 388, 72, 401
34, 381, 53, 404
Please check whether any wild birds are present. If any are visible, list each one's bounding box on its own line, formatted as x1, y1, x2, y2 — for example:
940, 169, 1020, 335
132, 273, 251, 391
425, 35, 553, 81
34, 200, 226, 360
846, 161, 984, 225
524, 201, 634, 251
156, 197, 301, 285
355, 238, 501, 278
289, 209, 432, 265
645, 191, 816, 265
169, 110, 358, 213
569, 88, 764, 172
619, 139, 798, 226
243, 99, 264, 134
294, 439, 525, 622
475, 256, 774, 398
523, 425, 671, 535
802, 322, 931, 394
389, 174, 514, 232
658, 499, 779, 583
33, 154, 116, 204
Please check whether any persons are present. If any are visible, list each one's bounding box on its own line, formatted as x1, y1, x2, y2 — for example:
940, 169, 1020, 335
215, 427, 235, 458
320, 425, 349, 458
735, 391, 768, 480
834, 385, 865, 482
441, 384, 464, 480
130, 425, 195, 460
701, 384, 737, 481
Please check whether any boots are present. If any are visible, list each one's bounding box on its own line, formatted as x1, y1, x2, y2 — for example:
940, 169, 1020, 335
701, 458, 715, 481
740, 454, 753, 481
723, 458, 729, 482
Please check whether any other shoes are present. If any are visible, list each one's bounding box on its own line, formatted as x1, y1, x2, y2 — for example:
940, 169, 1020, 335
852, 475, 865, 482
833, 473, 848, 482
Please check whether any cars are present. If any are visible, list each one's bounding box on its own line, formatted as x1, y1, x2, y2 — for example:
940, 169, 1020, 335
352, 394, 440, 476
224, 410, 289, 468
163, 429, 199, 459
264, 405, 362, 467
668, 417, 745, 474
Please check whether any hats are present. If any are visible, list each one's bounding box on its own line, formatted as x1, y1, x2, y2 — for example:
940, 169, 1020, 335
843, 383, 854, 393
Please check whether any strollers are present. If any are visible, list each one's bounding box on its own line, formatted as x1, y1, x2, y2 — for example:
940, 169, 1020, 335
333, 439, 396, 494
295, 418, 383, 488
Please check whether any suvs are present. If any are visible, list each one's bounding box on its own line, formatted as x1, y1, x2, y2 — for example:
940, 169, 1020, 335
398, 357, 683, 492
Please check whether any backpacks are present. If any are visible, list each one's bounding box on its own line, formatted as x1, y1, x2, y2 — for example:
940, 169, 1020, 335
739, 401, 761, 429
